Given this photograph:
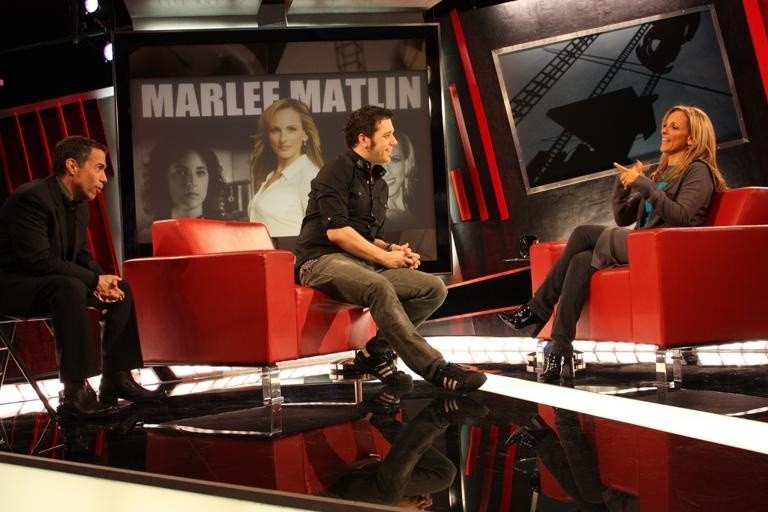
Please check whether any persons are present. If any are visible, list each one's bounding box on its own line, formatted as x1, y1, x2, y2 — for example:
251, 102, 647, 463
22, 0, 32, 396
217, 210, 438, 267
496, 106, 727, 383
339, 392, 639, 511
0, 136, 165, 408
294, 107, 487, 392
140, 99, 416, 238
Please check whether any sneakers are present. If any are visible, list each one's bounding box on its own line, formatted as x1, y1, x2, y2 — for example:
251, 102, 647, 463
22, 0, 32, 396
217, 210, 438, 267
355, 385, 414, 421
433, 363, 487, 393
353, 350, 412, 385
435, 394, 489, 423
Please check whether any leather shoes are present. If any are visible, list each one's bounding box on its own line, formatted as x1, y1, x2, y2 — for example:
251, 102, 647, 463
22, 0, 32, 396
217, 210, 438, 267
99, 371, 161, 404
57, 419, 114, 442
64, 388, 120, 418
113, 405, 168, 431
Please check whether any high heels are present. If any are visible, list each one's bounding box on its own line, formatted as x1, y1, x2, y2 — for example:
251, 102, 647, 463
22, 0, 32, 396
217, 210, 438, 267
504, 415, 550, 450
497, 305, 546, 338
538, 352, 576, 381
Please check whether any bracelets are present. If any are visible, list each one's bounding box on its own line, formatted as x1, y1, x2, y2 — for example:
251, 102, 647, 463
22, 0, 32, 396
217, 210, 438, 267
386, 243, 395, 252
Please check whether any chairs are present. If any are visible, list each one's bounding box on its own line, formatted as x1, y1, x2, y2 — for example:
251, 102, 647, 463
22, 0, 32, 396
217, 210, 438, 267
0, 306, 98, 422
525, 185, 768, 391
121, 216, 381, 408
